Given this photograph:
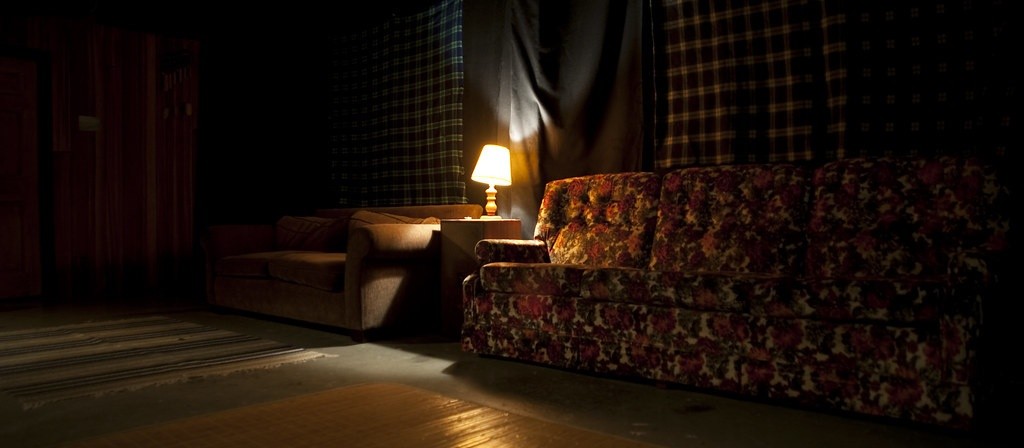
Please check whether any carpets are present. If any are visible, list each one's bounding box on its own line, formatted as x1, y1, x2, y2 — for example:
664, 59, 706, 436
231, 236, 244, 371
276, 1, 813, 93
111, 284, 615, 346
0, 314, 309, 413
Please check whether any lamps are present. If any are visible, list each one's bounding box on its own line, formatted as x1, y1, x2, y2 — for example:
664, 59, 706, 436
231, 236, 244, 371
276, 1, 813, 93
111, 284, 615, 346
470, 144, 512, 220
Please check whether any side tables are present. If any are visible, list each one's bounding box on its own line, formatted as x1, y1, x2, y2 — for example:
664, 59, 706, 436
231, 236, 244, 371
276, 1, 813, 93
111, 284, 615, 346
440, 218, 522, 339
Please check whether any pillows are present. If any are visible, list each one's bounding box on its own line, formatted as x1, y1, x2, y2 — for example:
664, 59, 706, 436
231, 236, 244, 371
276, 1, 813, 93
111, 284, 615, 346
269, 216, 342, 250
349, 212, 442, 240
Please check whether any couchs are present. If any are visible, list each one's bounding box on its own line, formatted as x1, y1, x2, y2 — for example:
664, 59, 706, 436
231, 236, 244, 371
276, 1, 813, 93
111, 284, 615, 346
199, 202, 483, 345
460, 155, 1012, 432
